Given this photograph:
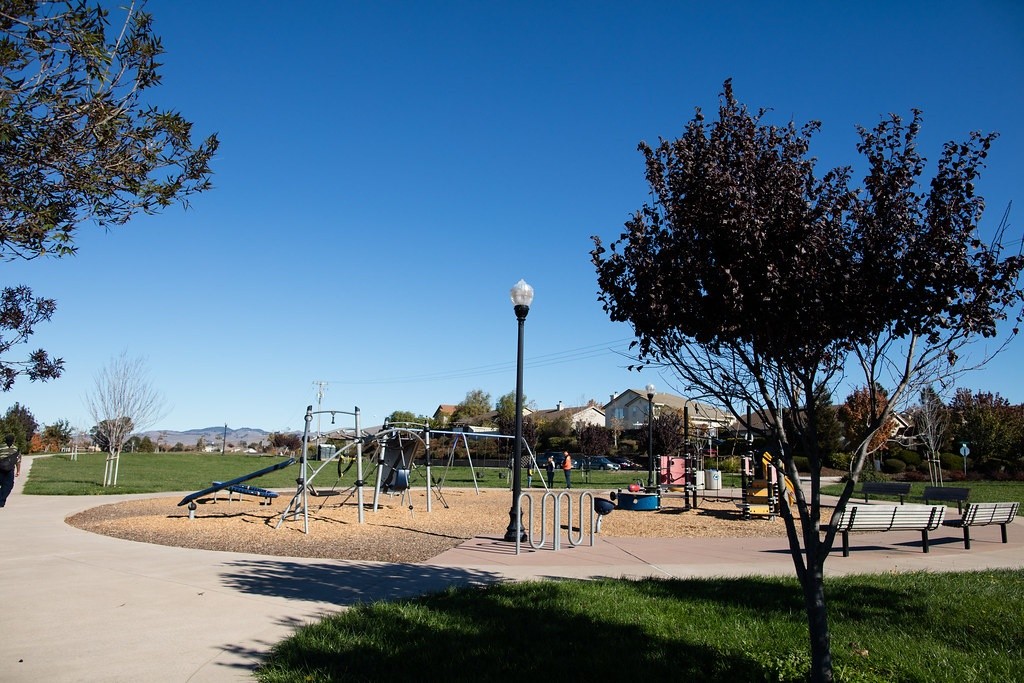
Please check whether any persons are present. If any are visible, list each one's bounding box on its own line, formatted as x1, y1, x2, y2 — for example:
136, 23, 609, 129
561, 451, 572, 488
527, 464, 533, 488
545, 456, 555, 488
0, 435, 21, 507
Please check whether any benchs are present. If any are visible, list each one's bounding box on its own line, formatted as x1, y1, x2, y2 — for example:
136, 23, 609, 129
820, 504, 948, 555
916, 486, 972, 515
852, 482, 912, 505
942, 502, 1020, 550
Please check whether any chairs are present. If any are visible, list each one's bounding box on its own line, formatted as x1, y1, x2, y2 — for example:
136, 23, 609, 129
385, 469, 411, 492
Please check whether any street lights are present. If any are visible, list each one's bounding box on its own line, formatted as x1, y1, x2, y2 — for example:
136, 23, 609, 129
503, 276, 534, 543
221, 421, 228, 456
642, 382, 658, 493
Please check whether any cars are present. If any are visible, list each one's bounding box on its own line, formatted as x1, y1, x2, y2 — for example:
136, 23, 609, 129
538, 451, 578, 469
605, 458, 642, 471
576, 456, 622, 471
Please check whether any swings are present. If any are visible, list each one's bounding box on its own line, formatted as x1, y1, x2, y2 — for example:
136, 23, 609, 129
475, 435, 488, 479
430, 432, 446, 485
497, 436, 510, 479
